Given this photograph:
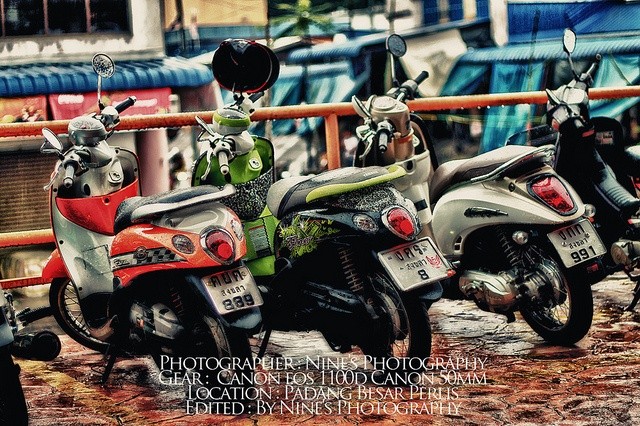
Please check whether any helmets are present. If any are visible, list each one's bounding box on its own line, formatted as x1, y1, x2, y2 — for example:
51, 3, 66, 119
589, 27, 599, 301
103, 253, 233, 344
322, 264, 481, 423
210, 37, 283, 95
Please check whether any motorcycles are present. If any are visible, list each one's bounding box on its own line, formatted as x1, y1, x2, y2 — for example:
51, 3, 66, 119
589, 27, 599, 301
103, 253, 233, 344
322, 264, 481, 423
504, 28, 640, 310
0, 301, 61, 426
349, 34, 610, 344
190, 37, 456, 386
39, 53, 264, 412
